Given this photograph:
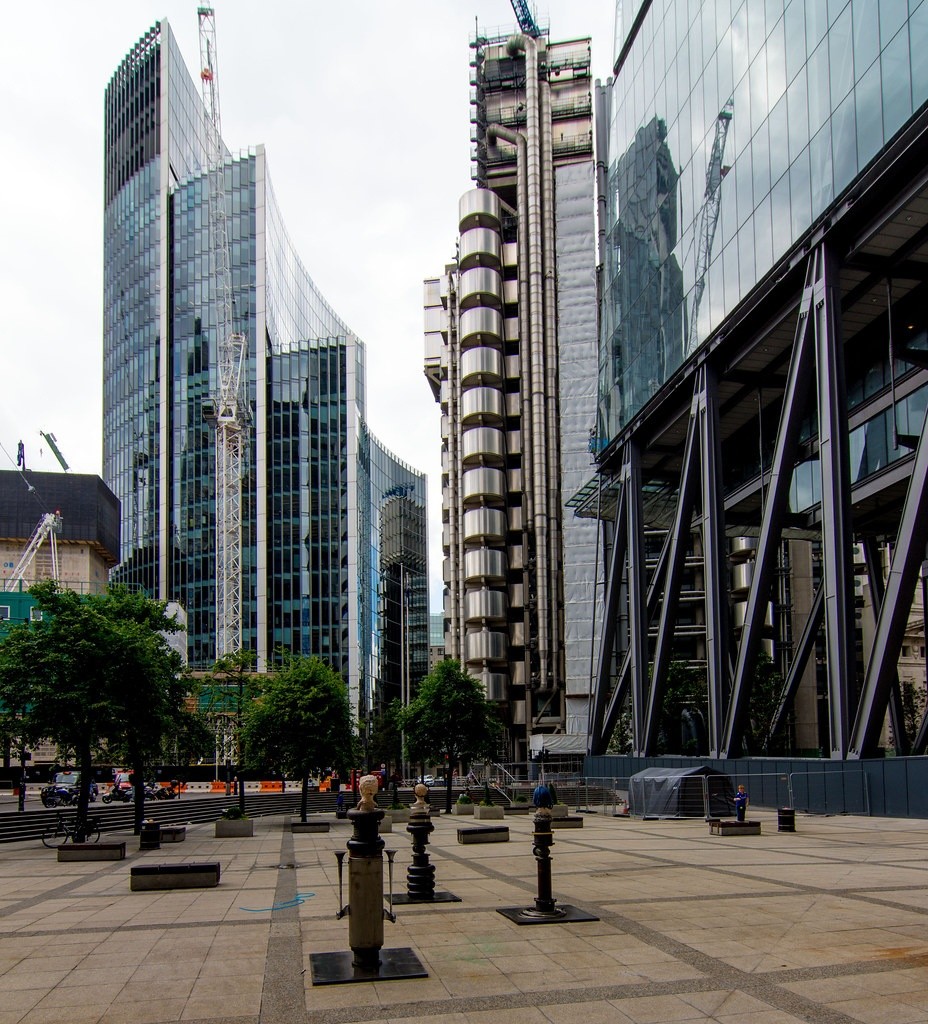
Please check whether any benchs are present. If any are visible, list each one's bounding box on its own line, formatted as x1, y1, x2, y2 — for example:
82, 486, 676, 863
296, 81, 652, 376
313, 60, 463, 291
129, 863, 221, 892
428, 808, 441, 816
708, 821, 762, 836
157, 826, 186, 843
550, 816, 584, 828
290, 822, 330, 833
456, 825, 510, 844
336, 811, 348, 819
56, 841, 126, 863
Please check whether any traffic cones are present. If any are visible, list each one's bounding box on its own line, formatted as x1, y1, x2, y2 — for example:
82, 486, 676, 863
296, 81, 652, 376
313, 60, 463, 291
623, 800, 628, 814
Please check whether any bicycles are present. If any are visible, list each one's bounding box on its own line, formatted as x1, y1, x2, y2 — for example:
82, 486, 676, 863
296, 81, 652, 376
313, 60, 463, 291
41, 811, 100, 848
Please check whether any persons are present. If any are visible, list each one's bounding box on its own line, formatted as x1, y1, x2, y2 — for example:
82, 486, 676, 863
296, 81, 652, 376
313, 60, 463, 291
533, 784, 552, 810
337, 791, 343, 811
734, 785, 750, 822
458, 788, 470, 798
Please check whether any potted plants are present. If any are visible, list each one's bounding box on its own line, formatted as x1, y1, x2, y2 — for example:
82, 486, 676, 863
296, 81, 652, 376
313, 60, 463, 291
216, 805, 254, 837
375, 781, 410, 833
451, 781, 568, 820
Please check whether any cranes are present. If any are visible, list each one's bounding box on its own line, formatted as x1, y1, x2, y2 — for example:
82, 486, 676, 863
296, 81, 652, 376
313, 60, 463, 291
510, 0, 541, 38
687, 98, 733, 356
198, 0, 248, 763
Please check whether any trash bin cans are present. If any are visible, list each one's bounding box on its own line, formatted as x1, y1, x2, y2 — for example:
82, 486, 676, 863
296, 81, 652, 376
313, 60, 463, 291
778, 807, 796, 833
331, 778, 340, 792
140, 823, 160, 850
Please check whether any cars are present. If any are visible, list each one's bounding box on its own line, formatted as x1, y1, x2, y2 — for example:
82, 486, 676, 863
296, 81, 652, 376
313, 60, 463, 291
417, 775, 435, 786
40, 771, 98, 806
111, 771, 134, 790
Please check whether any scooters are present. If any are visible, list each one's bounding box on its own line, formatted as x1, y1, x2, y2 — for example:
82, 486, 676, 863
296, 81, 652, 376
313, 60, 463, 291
43, 785, 81, 808
144, 780, 179, 801
102, 779, 133, 804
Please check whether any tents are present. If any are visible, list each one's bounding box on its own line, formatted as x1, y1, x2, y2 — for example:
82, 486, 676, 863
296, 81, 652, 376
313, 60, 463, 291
628, 765, 735, 819
